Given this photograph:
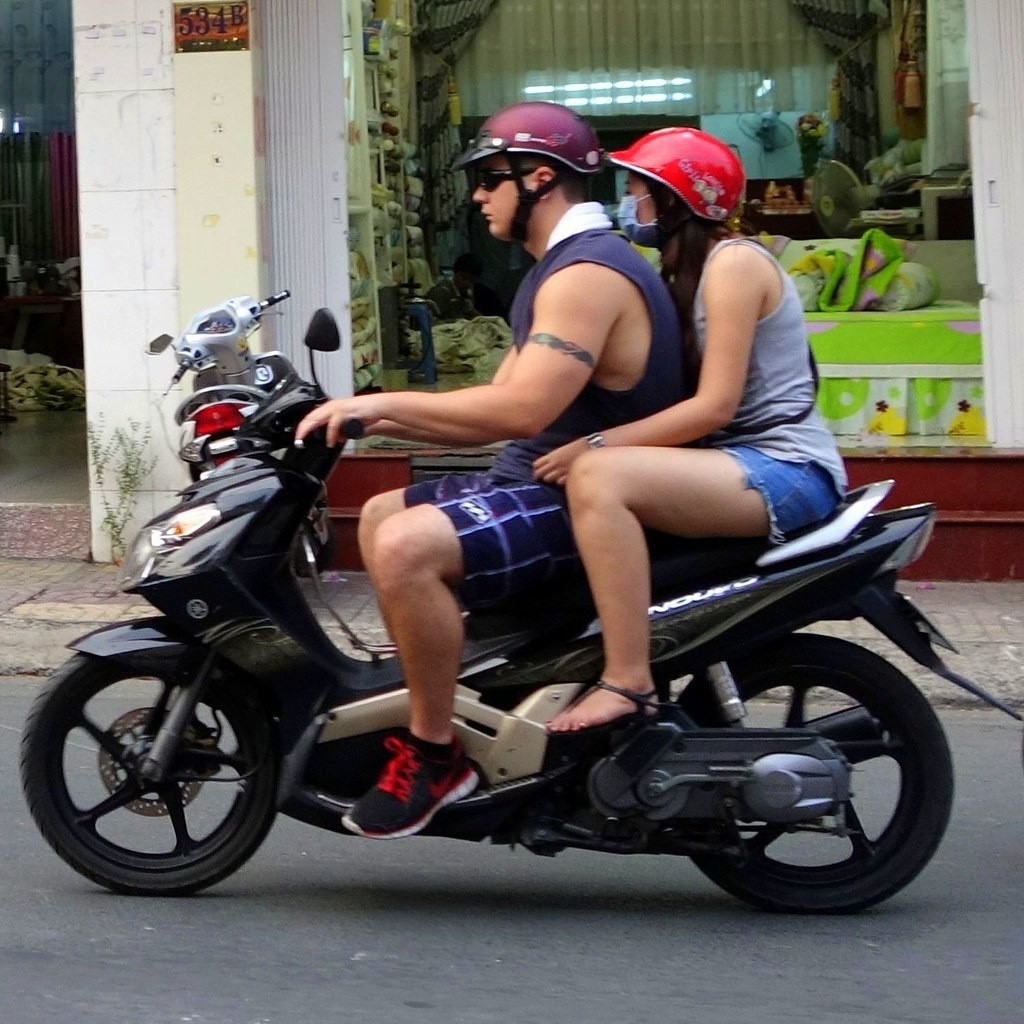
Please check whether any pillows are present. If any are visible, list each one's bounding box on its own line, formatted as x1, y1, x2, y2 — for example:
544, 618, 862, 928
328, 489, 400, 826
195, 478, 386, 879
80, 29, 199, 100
870, 261, 940, 311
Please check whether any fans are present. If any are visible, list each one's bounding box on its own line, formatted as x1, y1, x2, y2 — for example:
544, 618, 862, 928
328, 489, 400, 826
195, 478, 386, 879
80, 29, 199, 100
737, 109, 794, 152
811, 160, 868, 238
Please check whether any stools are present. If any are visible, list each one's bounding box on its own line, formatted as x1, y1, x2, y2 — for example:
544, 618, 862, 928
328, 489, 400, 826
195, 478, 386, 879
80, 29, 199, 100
400, 305, 438, 385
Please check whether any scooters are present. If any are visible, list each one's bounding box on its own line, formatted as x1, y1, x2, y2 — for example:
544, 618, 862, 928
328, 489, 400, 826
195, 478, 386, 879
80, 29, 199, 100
145, 289, 334, 578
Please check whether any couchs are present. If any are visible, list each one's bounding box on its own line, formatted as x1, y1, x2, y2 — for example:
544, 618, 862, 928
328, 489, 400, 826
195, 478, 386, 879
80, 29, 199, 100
752, 235, 986, 436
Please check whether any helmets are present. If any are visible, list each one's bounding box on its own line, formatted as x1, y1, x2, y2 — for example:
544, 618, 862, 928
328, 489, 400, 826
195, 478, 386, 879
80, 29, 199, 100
451, 102, 605, 173
609, 127, 744, 221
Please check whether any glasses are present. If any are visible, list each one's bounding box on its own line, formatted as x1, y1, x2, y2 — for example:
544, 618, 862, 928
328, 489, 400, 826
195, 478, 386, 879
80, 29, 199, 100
476, 167, 560, 191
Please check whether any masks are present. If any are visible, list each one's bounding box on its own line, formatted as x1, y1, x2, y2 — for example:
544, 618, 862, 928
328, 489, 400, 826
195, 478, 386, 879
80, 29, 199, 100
617, 194, 667, 246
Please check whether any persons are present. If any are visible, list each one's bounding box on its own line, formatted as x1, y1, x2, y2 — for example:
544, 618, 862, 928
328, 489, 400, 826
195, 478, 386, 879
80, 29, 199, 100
533, 126, 846, 731
297, 104, 686, 840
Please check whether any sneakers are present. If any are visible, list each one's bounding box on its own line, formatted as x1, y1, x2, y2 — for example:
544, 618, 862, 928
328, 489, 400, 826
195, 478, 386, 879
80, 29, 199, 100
341, 736, 479, 840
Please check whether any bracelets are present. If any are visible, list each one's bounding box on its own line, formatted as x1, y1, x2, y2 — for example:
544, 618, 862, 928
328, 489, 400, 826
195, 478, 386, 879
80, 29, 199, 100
586, 433, 606, 449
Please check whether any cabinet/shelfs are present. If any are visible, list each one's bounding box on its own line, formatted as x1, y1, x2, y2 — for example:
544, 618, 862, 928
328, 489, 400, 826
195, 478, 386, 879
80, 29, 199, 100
342, 0, 412, 391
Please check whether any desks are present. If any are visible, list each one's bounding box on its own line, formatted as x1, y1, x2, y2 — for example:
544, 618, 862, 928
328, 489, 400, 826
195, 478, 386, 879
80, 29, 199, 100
924, 185, 972, 240
743, 203, 815, 240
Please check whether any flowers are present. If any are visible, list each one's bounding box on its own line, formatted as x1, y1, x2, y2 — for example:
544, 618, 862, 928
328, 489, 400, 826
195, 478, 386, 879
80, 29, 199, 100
796, 112, 828, 177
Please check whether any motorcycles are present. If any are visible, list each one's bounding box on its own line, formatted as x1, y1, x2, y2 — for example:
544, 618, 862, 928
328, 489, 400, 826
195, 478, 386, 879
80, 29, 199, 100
18, 307, 1023, 915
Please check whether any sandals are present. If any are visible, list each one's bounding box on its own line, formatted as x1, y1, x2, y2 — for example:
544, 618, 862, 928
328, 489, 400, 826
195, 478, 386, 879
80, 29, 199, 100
546, 681, 683, 740
